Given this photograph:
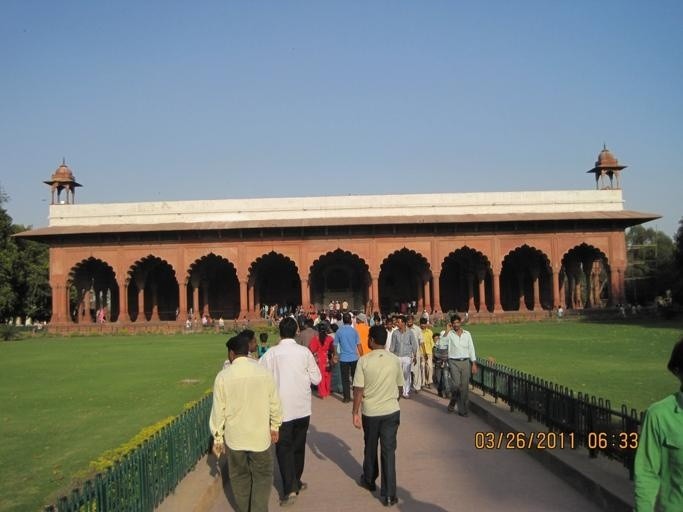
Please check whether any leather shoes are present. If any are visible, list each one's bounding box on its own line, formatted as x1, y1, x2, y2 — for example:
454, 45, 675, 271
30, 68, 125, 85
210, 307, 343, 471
385, 496, 398, 506
361, 475, 375, 490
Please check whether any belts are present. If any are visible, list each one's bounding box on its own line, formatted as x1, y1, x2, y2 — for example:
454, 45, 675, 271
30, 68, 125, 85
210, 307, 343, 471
450, 359, 468, 361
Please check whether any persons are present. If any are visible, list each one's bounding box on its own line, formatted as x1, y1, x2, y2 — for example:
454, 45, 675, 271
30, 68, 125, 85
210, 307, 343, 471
257, 316, 323, 507
209, 336, 282, 512
558, 305, 564, 322
353, 325, 404, 506
96, 306, 109, 324
615, 289, 672, 320
634, 338, 683, 512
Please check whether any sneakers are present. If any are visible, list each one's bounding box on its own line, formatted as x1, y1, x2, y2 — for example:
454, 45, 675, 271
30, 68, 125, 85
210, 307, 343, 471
279, 492, 298, 507
447, 399, 456, 412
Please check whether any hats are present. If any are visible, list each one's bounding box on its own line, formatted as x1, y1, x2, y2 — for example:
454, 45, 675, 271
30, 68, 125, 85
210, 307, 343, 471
356, 313, 366, 321
